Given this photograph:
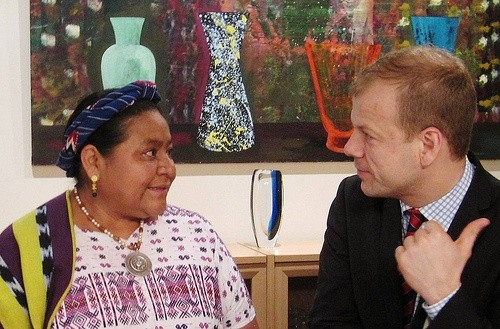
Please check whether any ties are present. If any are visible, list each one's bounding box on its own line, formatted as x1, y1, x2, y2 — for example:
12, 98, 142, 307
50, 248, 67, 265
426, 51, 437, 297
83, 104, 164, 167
403, 208, 429, 327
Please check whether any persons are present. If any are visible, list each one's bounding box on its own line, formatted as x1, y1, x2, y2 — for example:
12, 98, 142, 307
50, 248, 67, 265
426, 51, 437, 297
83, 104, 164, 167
307, 47, 500, 329
0, 82, 261, 329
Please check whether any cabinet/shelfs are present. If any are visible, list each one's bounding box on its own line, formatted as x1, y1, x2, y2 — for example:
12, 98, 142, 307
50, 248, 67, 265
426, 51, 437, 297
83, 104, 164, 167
221, 236, 324, 328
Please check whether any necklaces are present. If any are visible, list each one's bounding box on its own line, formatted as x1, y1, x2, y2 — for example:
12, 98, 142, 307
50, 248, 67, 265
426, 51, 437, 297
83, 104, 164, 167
73, 185, 151, 276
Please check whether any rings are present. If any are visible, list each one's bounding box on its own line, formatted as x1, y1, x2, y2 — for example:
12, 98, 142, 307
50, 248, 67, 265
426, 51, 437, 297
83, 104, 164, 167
419, 222, 427, 229
